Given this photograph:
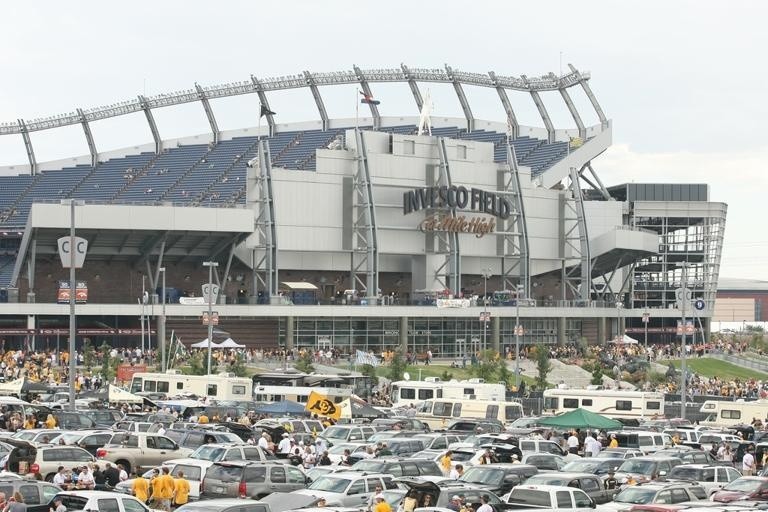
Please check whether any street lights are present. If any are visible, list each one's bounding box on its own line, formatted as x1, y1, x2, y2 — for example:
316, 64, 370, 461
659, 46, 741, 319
157, 259, 221, 379
479, 268, 524, 372
614, 294, 625, 357
57, 197, 87, 412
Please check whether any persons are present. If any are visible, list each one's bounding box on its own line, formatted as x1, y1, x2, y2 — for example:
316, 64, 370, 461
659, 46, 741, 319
0, 336, 768, 512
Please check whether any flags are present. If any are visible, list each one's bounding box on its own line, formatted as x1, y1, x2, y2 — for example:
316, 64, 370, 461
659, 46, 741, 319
360, 91, 382, 104
570, 136, 584, 149
260, 105, 277, 118
